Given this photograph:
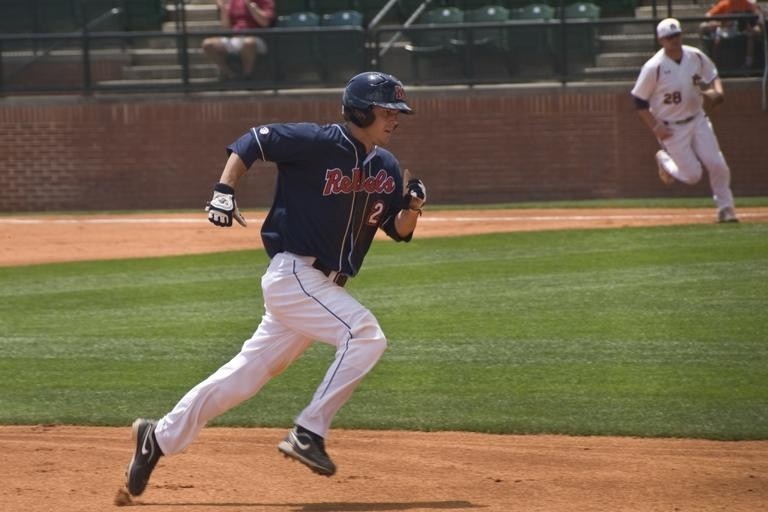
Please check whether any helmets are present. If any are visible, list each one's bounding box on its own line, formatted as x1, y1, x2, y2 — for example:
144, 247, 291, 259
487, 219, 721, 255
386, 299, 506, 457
341, 72, 415, 115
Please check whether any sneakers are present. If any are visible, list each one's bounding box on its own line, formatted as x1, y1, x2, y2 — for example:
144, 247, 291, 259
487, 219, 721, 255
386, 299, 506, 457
278, 426, 336, 477
656, 150, 675, 184
718, 207, 738, 223
126, 418, 163, 495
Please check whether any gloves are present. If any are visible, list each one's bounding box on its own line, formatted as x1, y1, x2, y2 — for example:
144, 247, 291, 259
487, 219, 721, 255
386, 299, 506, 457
403, 169, 426, 214
205, 184, 247, 228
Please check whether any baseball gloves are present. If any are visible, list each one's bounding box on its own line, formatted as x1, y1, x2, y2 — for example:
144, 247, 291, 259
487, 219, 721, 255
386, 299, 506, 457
698, 89, 723, 117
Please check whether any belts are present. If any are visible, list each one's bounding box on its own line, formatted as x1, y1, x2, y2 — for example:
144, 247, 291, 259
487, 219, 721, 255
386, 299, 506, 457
663, 116, 694, 125
312, 258, 348, 286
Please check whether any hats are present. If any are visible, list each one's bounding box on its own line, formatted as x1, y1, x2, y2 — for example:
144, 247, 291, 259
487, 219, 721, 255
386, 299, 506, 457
657, 18, 682, 41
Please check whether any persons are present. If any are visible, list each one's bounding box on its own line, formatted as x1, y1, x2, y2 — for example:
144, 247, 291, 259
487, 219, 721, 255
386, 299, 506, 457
698, 0, 767, 74
127, 70, 427, 498
627, 16, 743, 227
200, 0, 281, 86
